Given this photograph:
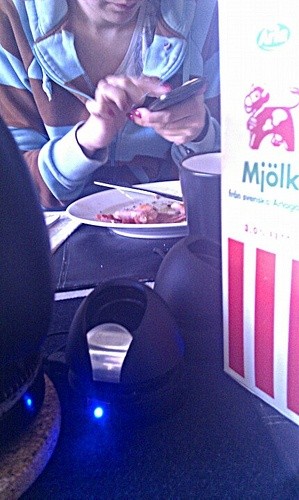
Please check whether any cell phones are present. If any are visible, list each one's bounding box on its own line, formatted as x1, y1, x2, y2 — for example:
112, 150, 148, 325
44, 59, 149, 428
147, 77, 206, 112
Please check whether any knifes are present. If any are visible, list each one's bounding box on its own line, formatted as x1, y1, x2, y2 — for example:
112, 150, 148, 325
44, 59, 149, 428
92, 174, 183, 209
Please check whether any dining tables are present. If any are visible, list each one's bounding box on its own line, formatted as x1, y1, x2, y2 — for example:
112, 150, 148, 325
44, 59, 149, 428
17, 208, 299, 500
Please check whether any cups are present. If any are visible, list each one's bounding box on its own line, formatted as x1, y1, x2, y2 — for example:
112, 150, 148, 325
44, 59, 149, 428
1, 116, 53, 452
179, 150, 225, 256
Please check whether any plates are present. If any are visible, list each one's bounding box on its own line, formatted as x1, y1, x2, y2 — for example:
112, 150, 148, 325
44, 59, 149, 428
66, 181, 190, 240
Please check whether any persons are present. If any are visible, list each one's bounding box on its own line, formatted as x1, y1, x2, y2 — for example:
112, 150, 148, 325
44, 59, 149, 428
0, 0, 221, 209
